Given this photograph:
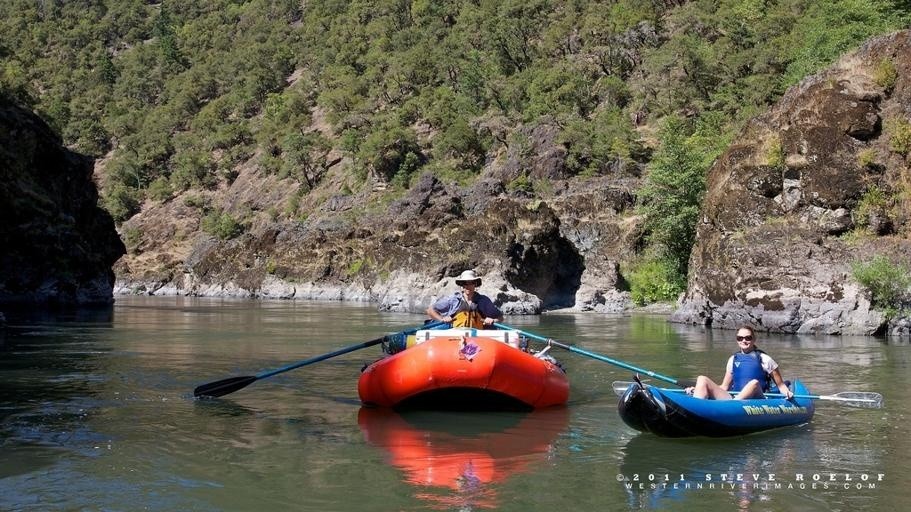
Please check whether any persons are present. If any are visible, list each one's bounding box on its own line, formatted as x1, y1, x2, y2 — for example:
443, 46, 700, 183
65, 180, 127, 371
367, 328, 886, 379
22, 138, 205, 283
426, 271, 504, 330
693, 326, 793, 400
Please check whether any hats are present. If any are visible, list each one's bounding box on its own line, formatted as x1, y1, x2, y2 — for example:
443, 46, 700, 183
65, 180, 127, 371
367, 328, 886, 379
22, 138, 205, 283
455, 269, 484, 288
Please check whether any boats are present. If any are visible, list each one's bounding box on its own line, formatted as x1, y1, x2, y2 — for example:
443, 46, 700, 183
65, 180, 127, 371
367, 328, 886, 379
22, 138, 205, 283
355, 409, 570, 511
614, 422, 817, 511
616, 374, 818, 438
353, 331, 572, 412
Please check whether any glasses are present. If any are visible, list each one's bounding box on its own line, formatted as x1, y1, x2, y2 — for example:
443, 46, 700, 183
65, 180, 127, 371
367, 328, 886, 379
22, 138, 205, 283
737, 334, 753, 342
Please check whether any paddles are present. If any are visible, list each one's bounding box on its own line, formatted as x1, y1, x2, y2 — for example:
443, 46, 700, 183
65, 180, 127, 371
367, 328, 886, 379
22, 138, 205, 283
612, 380, 885, 410
479, 319, 695, 386
194, 316, 457, 398
